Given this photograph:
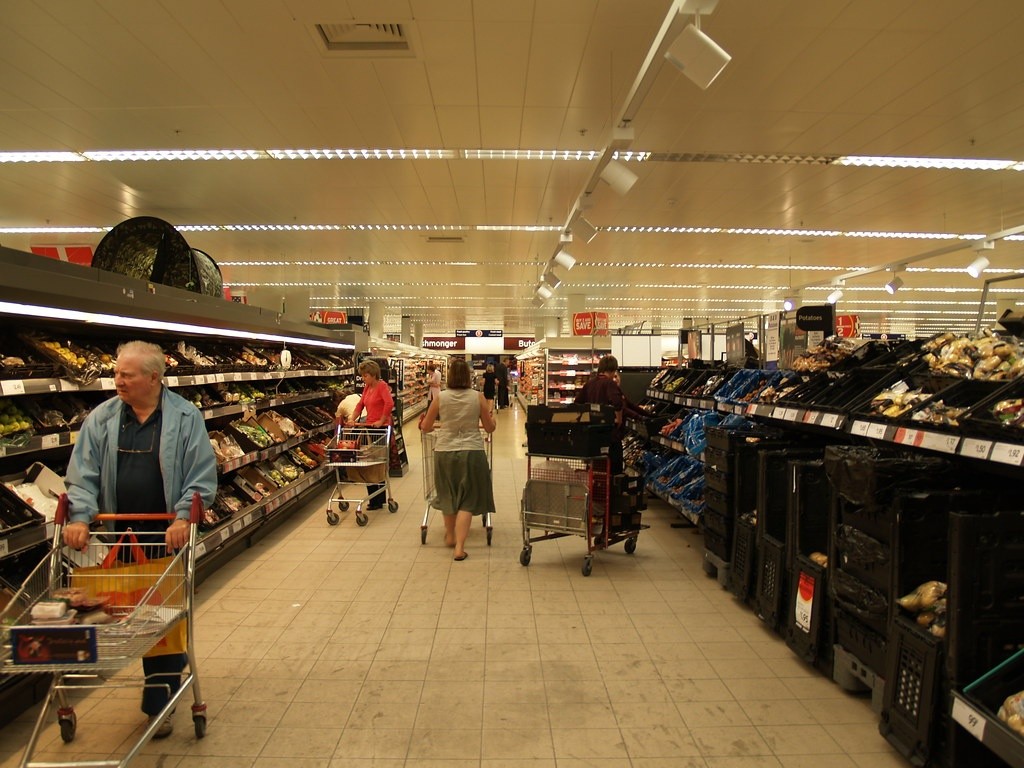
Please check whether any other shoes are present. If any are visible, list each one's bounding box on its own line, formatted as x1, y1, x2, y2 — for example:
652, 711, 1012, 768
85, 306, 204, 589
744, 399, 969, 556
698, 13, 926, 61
148, 715, 172, 737
367, 504, 382, 510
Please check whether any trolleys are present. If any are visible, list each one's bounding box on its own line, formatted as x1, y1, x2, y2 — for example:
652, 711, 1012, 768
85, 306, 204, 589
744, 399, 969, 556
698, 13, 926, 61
1, 490, 208, 768
519, 453, 650, 576
479, 381, 500, 413
418, 421, 495, 546
322, 413, 399, 527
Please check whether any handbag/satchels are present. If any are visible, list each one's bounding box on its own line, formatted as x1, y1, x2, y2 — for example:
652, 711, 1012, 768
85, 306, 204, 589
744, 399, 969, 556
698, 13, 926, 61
70, 529, 187, 658
346, 427, 386, 483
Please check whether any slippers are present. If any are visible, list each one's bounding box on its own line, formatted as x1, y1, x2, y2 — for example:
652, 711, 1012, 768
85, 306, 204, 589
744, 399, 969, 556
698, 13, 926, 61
455, 551, 468, 561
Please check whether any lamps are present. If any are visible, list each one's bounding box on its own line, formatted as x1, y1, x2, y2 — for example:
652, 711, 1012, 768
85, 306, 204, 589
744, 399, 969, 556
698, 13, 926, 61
784, 299, 802, 312
554, 250, 577, 272
537, 285, 553, 301
966, 251, 990, 279
531, 297, 547, 311
542, 267, 562, 290
598, 159, 640, 199
571, 211, 599, 244
663, 9, 732, 90
827, 286, 844, 304
885, 271, 903, 294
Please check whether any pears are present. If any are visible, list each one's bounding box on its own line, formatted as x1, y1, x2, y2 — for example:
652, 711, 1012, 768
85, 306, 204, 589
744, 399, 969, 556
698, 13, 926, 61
42, 341, 86, 367
270, 470, 289, 487
230, 383, 264, 401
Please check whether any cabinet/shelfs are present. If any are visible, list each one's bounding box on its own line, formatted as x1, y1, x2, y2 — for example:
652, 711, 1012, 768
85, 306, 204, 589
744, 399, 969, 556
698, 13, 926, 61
517, 347, 611, 411
377, 351, 451, 425
632, 338, 1024, 768
0, 245, 368, 730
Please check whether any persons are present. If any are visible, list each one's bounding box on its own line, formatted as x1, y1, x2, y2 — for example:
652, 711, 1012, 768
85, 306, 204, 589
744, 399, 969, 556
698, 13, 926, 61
422, 364, 441, 420
346, 360, 393, 509
421, 359, 496, 561
333, 389, 367, 423
575, 355, 648, 547
62, 340, 218, 737
495, 358, 509, 409
483, 364, 495, 417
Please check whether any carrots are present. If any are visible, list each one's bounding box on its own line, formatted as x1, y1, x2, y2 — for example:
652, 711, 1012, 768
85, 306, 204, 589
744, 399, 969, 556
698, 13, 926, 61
793, 345, 832, 372
661, 418, 683, 435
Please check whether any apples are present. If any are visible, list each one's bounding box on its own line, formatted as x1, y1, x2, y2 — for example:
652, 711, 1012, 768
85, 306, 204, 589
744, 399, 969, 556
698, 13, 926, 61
294, 446, 317, 465
0, 398, 34, 434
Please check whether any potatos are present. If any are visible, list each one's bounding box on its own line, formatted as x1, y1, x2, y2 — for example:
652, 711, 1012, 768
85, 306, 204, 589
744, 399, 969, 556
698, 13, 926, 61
920, 332, 1024, 381
897, 579, 947, 638
996, 690, 1024, 738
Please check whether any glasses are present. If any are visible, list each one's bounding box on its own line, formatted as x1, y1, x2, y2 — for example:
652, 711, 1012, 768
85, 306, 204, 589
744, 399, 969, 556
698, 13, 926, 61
116, 425, 156, 453
